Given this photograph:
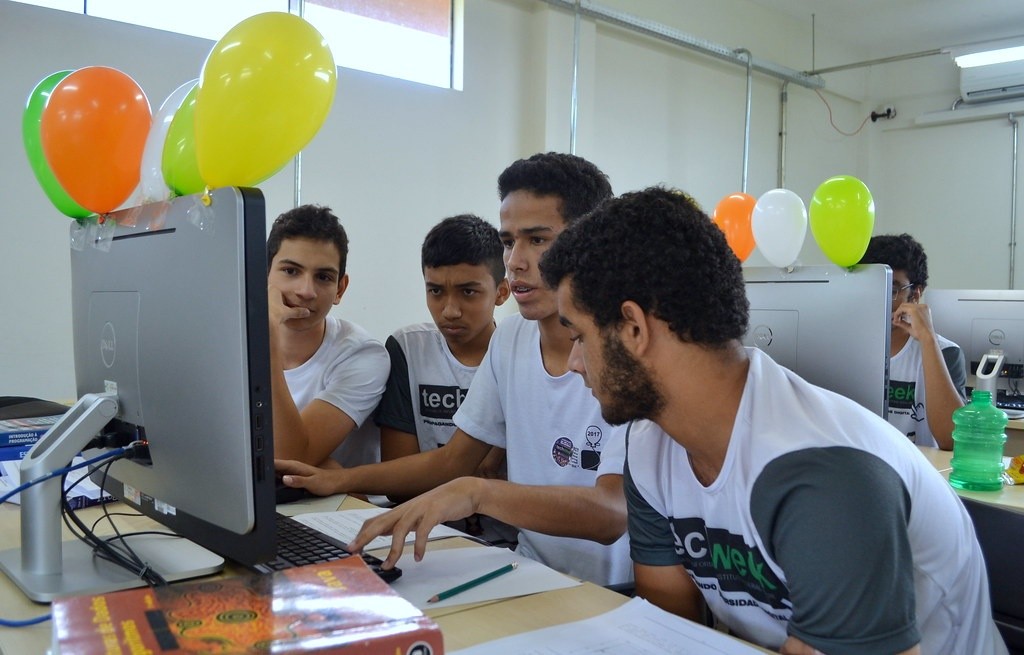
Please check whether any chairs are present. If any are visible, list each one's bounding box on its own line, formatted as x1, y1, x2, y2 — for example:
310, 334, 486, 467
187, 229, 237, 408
957, 497, 1023, 655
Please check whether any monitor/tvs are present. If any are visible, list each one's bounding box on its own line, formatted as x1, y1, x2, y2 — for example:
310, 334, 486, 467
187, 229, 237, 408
0, 186, 279, 604
921, 290, 1024, 420
737, 264, 893, 423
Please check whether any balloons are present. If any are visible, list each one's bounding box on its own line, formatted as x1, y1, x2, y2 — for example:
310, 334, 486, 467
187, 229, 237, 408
713, 174, 877, 269
23, 11, 338, 225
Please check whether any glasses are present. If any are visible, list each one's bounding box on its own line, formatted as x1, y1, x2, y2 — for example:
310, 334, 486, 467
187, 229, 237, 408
893, 284, 914, 300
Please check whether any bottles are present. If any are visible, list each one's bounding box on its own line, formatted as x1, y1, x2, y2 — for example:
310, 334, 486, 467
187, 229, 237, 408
948, 390, 1009, 492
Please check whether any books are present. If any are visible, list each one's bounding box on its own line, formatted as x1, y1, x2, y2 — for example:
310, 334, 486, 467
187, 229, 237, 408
50, 552, 445, 655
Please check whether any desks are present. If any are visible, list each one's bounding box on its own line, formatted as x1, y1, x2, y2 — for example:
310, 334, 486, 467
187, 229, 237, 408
0, 494, 783, 655
918, 447, 1024, 519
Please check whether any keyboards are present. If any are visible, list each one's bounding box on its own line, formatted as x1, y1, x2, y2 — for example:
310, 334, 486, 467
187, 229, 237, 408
250, 511, 402, 584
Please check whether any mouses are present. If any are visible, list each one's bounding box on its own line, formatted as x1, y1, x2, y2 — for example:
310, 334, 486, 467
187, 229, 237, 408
274, 474, 304, 504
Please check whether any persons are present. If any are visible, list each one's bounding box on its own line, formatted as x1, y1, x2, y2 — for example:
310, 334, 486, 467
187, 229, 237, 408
539, 189, 1013, 655
266, 205, 392, 502
860, 234, 968, 453
376, 215, 521, 552
272, 150, 635, 597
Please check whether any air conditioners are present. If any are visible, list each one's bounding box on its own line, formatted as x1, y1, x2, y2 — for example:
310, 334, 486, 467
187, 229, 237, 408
960, 59, 1024, 104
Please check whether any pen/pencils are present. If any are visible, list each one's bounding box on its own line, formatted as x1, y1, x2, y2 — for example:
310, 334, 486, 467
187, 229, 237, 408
428, 562, 519, 603
